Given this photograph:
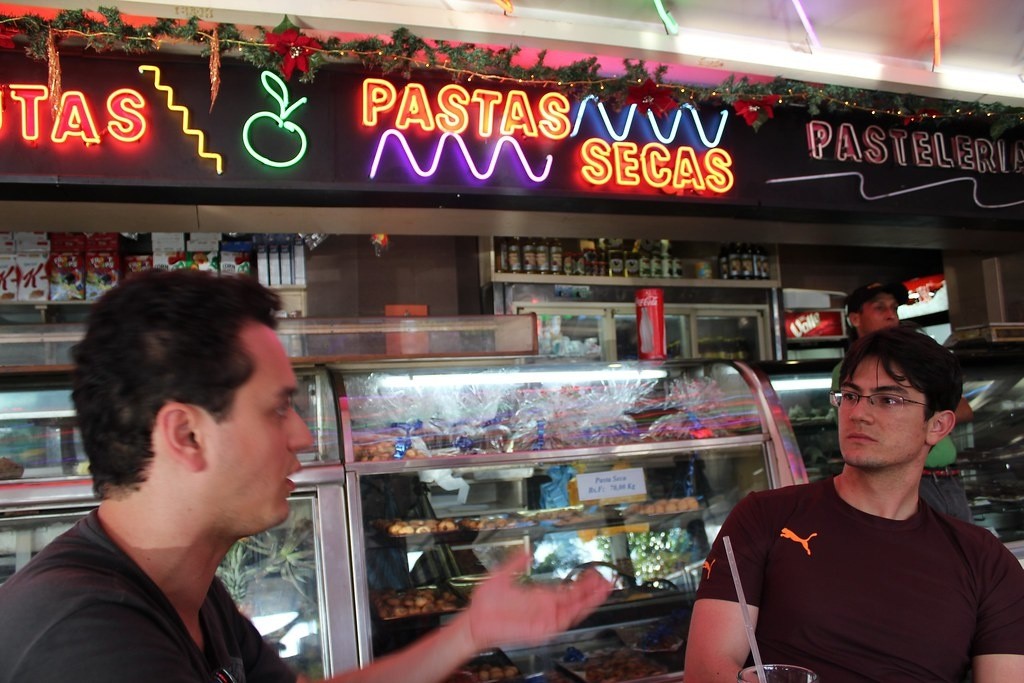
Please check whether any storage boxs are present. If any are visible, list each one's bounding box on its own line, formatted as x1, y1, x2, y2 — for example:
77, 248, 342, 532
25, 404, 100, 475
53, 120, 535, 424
0, 232, 255, 304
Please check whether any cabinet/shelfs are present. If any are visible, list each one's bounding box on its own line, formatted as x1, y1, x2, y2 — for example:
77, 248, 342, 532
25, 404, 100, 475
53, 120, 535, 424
0, 351, 1024, 683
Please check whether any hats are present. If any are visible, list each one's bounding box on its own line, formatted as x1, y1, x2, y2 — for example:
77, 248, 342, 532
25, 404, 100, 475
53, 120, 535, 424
843, 281, 908, 328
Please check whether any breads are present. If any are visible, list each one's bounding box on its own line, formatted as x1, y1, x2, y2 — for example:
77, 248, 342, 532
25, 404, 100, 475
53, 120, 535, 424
350, 433, 699, 683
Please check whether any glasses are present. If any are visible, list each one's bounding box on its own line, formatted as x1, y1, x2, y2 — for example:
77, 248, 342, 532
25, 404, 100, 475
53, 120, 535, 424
829, 389, 927, 411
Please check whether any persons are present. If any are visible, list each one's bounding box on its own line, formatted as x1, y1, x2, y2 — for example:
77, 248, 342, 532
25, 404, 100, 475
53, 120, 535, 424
0, 268, 613, 683
685, 328, 1024, 683
831, 283, 973, 520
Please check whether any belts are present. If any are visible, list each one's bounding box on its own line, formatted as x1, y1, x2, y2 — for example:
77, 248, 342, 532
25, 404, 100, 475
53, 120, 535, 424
923, 466, 960, 483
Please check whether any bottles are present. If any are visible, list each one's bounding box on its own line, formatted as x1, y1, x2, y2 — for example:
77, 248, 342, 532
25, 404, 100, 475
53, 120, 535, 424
494, 236, 682, 276
668, 337, 748, 359
717, 243, 770, 281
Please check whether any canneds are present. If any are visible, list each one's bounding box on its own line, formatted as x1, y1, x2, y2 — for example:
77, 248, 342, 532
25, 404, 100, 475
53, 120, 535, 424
563, 247, 609, 276
635, 286, 668, 360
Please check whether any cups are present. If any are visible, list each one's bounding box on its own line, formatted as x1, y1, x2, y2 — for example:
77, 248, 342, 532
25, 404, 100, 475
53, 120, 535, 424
736, 664, 820, 683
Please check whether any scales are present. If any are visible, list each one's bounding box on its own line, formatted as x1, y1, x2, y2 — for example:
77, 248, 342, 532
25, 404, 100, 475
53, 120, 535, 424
953, 322, 1024, 351
